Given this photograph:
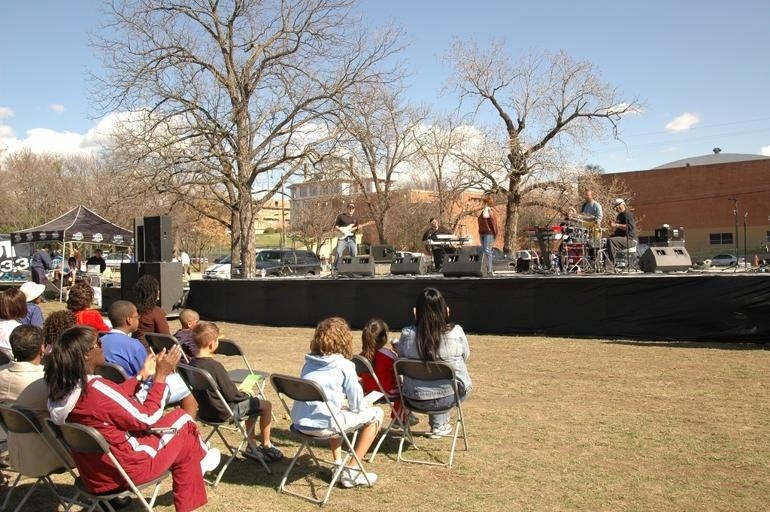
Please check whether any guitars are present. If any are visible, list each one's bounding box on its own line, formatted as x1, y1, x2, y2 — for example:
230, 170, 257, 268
338, 220, 376, 240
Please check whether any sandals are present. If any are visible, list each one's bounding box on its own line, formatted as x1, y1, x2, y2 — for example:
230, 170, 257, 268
241, 444, 271, 463
257, 442, 284, 459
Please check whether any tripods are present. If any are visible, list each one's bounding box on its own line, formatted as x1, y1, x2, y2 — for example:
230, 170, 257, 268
722, 203, 751, 273
593, 231, 615, 272
568, 218, 594, 272
619, 204, 638, 273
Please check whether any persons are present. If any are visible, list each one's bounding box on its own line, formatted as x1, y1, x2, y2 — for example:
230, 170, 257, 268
558, 207, 578, 274
397, 287, 473, 439
579, 191, 603, 225
47, 325, 222, 512
477, 196, 499, 277
331, 203, 363, 277
173, 308, 200, 363
14, 281, 46, 330
31, 244, 54, 303
290, 316, 385, 488
86, 248, 107, 274
421, 217, 454, 271
180, 249, 190, 280
67, 282, 112, 332
98, 299, 200, 421
0, 288, 28, 363
42, 310, 77, 354
607, 198, 639, 272
68, 257, 77, 285
131, 274, 172, 356
357, 318, 420, 429
188, 323, 283, 462
0, 324, 46, 410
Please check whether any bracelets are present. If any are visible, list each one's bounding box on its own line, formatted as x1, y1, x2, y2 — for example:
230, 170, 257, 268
615, 224, 617, 228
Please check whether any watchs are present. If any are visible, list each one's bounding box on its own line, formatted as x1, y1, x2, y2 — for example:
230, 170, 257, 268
134, 374, 146, 384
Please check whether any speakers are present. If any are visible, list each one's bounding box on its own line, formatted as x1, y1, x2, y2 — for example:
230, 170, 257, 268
134, 216, 144, 262
390, 256, 428, 275
441, 253, 489, 278
141, 261, 184, 313
143, 216, 173, 262
120, 263, 140, 307
336, 255, 376, 277
638, 247, 693, 274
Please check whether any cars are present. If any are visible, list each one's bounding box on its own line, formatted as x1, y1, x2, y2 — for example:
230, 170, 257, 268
232, 250, 321, 276
711, 254, 745, 267
203, 248, 273, 280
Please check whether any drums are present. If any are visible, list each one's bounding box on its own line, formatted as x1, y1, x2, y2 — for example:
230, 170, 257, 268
560, 242, 585, 271
562, 226, 591, 241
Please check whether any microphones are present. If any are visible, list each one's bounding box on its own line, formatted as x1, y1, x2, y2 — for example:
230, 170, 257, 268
565, 197, 572, 207
744, 211, 749, 217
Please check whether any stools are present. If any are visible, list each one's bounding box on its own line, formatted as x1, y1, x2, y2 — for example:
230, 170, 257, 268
621, 246, 639, 273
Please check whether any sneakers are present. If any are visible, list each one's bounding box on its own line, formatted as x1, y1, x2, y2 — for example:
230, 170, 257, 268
431, 424, 453, 435
200, 448, 222, 476
392, 413, 420, 428
340, 469, 377, 488
330, 464, 342, 483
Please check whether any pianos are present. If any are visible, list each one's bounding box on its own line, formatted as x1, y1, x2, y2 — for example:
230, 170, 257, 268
424, 234, 473, 246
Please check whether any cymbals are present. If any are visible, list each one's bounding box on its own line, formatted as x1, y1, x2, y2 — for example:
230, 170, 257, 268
594, 228, 608, 230
571, 213, 595, 218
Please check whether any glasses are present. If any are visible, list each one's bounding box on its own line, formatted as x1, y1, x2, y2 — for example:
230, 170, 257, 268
88, 336, 102, 350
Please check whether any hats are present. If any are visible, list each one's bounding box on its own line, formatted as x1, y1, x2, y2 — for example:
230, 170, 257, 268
347, 203, 355, 209
614, 198, 625, 205
20, 281, 46, 303
